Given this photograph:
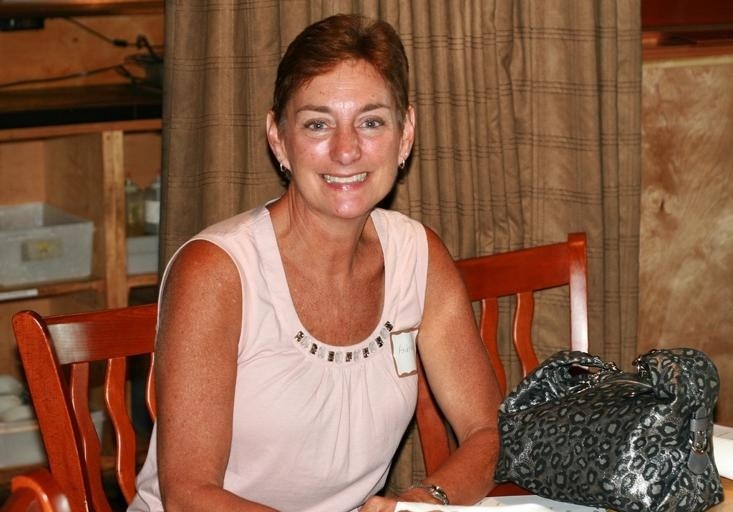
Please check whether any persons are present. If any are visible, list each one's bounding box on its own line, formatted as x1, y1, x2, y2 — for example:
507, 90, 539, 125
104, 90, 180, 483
119, 12, 510, 512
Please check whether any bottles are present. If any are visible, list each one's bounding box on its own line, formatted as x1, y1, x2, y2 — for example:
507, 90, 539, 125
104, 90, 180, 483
143, 175, 162, 235
123, 177, 141, 226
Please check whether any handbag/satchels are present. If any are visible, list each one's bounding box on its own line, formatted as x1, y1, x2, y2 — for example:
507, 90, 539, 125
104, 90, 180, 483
495, 348, 725, 512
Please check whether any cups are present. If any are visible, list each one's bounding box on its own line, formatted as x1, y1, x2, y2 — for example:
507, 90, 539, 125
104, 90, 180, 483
0, 374, 33, 423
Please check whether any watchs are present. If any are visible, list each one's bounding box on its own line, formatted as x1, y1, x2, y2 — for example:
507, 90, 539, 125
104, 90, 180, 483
408, 481, 450, 508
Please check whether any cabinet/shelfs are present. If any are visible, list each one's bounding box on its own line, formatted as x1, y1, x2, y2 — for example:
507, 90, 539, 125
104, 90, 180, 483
0, 119, 173, 490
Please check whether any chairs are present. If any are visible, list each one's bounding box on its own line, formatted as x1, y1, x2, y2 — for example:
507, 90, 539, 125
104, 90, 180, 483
11, 300, 249, 511
414, 231, 590, 497
